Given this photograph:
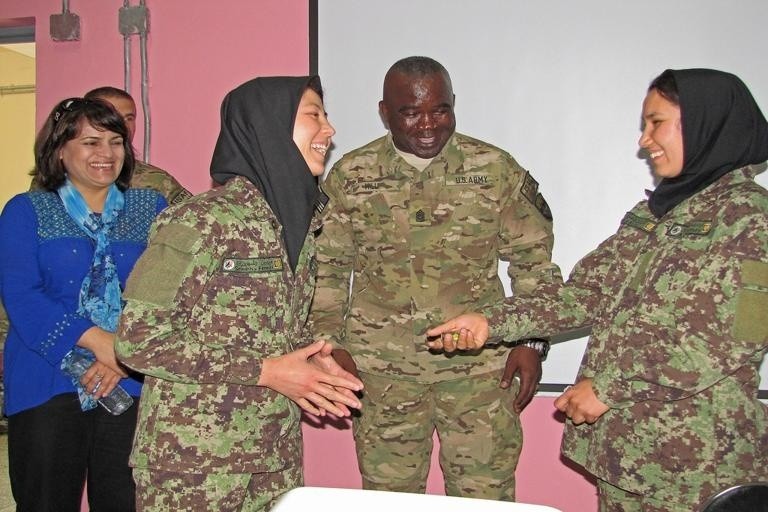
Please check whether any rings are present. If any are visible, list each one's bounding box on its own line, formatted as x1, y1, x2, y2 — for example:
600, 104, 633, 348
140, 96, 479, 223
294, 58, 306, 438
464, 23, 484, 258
95, 374, 103, 380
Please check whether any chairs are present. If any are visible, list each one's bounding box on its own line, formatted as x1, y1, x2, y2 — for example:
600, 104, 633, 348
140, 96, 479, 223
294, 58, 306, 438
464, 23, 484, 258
693, 477, 767, 512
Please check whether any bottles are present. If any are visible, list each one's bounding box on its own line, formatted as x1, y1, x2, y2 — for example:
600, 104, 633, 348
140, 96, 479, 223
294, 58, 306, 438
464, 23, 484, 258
64, 349, 135, 416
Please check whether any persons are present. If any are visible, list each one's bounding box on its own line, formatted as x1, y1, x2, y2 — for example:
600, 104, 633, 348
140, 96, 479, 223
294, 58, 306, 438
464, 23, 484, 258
114, 76, 365, 512
1, 85, 195, 433
1, 96, 166, 512
427, 68, 768, 512
303, 57, 564, 502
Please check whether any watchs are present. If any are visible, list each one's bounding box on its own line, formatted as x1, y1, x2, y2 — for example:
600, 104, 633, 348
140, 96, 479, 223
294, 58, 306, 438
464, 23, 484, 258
519, 338, 549, 360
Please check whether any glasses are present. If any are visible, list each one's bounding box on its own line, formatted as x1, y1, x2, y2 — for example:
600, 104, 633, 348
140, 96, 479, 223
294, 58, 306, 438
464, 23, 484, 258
53, 98, 114, 125
